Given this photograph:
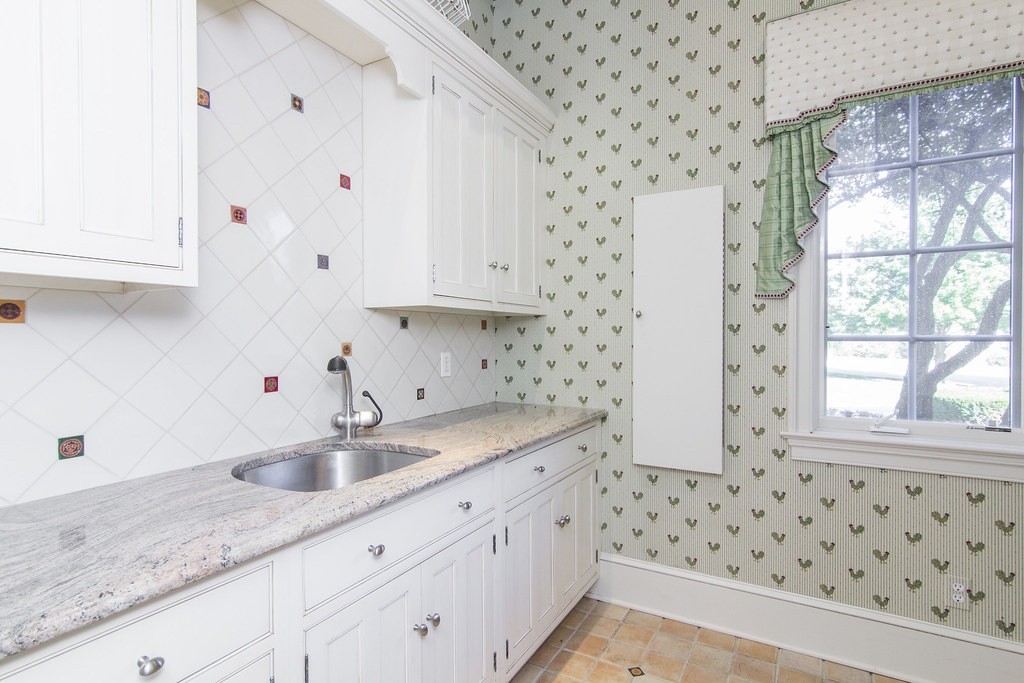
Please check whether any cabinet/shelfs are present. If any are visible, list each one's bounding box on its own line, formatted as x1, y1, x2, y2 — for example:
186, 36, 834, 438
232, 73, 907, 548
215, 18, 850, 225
360, 60, 546, 317
0, 421, 601, 683
0, 0, 199, 295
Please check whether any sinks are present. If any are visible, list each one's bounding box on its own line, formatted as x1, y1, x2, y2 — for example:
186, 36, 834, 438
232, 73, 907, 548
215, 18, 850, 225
230, 441, 441, 493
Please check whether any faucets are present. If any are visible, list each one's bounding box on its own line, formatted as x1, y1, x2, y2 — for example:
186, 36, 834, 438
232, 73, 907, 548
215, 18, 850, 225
326, 355, 377, 438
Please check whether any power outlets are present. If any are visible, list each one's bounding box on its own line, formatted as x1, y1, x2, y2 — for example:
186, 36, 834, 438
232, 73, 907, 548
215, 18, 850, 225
440, 352, 451, 376
